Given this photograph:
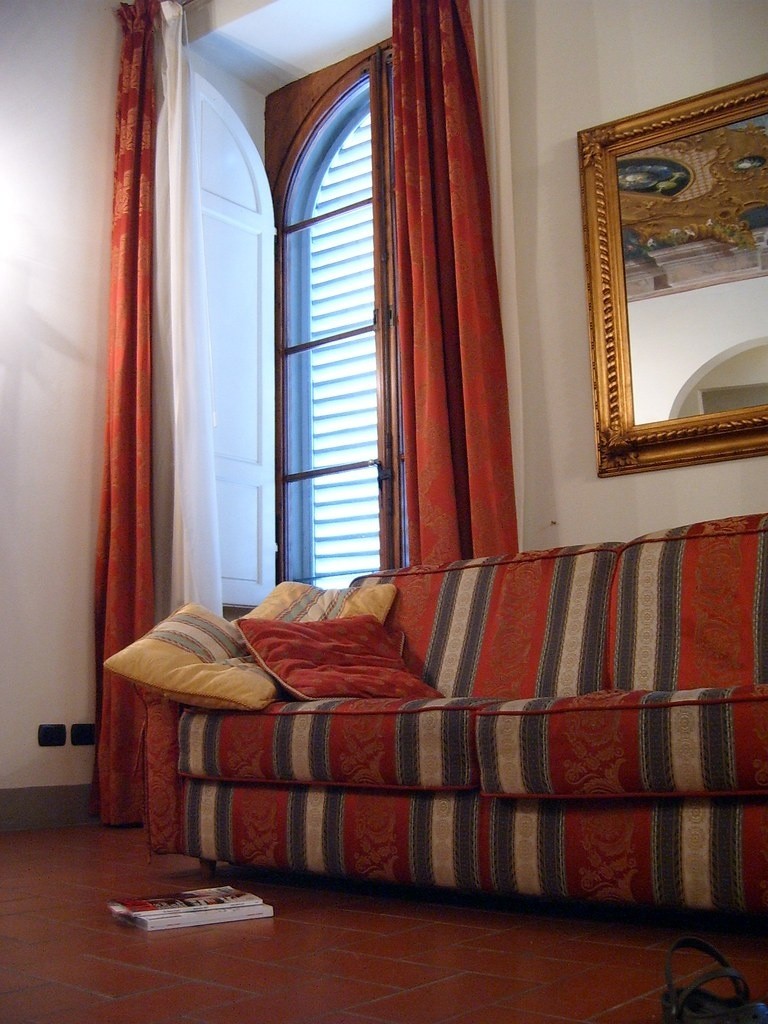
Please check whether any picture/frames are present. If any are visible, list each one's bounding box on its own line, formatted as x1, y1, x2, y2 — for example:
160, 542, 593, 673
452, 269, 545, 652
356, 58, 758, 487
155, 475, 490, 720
576, 72, 768, 478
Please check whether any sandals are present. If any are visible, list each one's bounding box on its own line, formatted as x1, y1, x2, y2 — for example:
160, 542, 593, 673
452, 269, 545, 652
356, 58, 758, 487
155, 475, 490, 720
661, 936, 768, 1024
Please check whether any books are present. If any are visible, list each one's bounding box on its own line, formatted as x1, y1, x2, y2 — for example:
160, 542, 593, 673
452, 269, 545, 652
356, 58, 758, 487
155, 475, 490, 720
109, 885, 264, 917
113, 904, 274, 931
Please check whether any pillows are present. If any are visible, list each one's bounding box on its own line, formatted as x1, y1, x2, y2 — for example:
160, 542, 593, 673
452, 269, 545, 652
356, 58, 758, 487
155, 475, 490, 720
235, 615, 446, 703
102, 603, 285, 711
230, 581, 398, 628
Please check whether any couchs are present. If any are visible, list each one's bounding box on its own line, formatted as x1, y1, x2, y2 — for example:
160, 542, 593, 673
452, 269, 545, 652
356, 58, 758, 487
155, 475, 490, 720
101, 513, 768, 927
